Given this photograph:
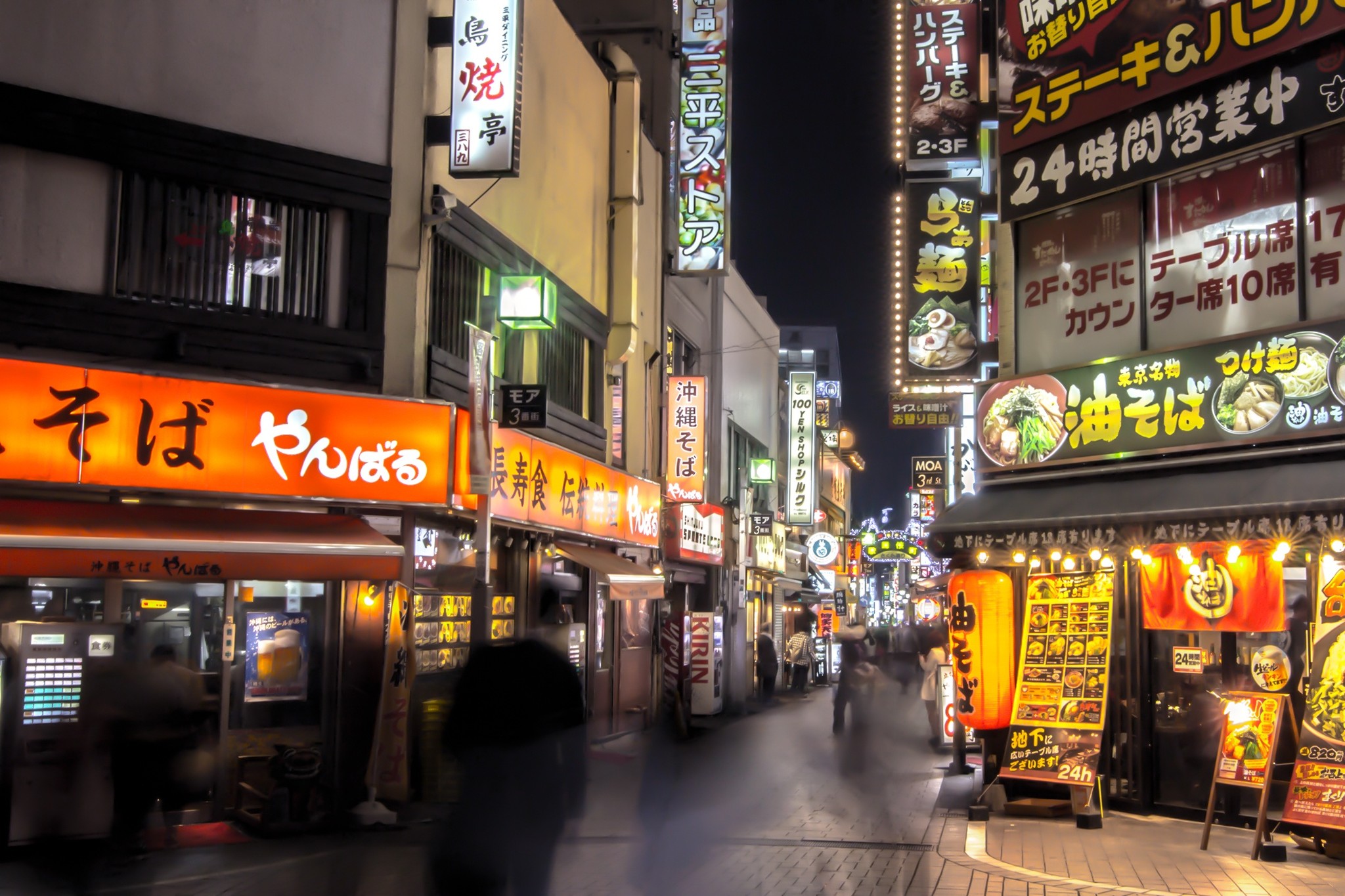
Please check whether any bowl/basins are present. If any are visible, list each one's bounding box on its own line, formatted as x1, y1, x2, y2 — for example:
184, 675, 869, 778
1046, 706, 1057, 717
1059, 700, 1084, 722
1211, 369, 1284, 441
1326, 335, 1345, 407
908, 322, 977, 370
977, 373, 1069, 467
1302, 620, 1345, 762
1284, 331, 1337, 410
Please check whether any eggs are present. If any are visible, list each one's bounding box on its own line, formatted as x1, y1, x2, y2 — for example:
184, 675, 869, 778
924, 308, 956, 329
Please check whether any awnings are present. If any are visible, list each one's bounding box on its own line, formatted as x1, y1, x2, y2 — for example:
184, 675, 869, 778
927, 445, 1345, 546
0, 497, 404, 582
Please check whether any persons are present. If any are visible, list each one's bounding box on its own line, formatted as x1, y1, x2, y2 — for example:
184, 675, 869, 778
427, 571, 592, 896
753, 618, 950, 751
120, 643, 208, 864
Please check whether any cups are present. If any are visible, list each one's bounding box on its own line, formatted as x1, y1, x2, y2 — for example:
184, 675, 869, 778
1167, 691, 1175, 702
274, 629, 299, 681
257, 640, 275, 682
1178, 697, 1184, 706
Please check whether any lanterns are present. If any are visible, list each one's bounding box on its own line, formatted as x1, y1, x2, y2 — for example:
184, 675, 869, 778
948, 569, 1015, 731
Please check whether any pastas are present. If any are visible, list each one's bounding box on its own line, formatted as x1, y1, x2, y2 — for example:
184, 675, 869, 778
1274, 345, 1328, 397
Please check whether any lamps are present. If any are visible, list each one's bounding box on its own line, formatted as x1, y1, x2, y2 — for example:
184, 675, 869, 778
819, 451, 866, 471
821, 420, 855, 448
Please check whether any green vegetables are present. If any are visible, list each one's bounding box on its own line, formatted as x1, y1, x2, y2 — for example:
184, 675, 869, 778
1006, 406, 1058, 462
1217, 404, 1238, 430
1245, 741, 1262, 760
907, 315, 931, 337
950, 322, 970, 336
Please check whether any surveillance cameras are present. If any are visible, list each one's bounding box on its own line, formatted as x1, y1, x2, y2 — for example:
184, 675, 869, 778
431, 193, 456, 211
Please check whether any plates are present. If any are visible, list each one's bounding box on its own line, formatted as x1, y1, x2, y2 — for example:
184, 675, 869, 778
492, 597, 513, 638
1030, 611, 1048, 628
415, 624, 438, 639
416, 650, 445, 668
1222, 751, 1243, 767
1064, 671, 1083, 688
416, 604, 438, 612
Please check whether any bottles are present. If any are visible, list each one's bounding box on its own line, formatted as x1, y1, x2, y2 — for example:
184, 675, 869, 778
438, 623, 464, 642
445, 598, 451, 616
453, 598, 458, 616
460, 598, 464, 618
440, 598, 444, 616
1209, 643, 1241, 665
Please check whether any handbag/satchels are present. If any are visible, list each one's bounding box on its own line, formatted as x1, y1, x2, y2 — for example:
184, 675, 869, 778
785, 635, 807, 662
